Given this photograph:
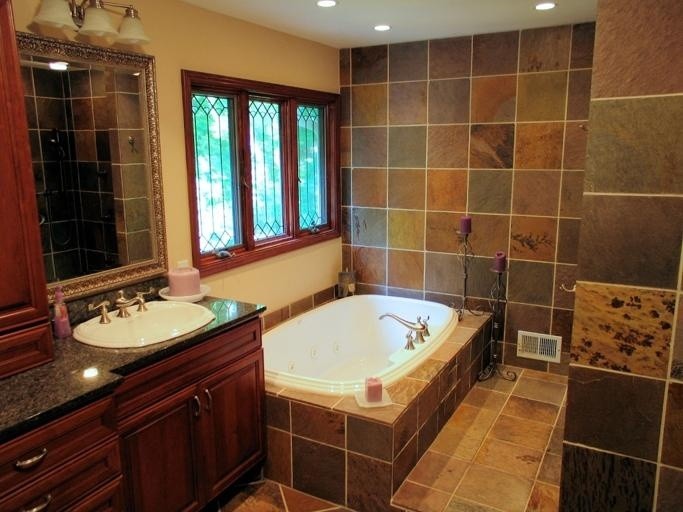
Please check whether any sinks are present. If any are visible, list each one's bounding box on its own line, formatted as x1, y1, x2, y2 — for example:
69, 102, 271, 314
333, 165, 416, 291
72, 301, 216, 349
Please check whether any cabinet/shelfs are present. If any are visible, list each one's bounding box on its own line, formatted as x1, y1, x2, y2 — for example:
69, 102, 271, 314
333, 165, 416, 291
1, 315, 266, 512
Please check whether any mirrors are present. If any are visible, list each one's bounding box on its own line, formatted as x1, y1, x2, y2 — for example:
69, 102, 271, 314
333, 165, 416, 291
15, 31, 169, 307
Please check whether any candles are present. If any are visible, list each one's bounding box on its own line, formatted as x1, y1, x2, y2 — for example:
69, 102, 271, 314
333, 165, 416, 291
494, 250, 506, 270
461, 216, 471, 233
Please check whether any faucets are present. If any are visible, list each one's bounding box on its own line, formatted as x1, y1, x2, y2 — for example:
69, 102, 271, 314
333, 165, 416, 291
115, 291, 145, 318
379, 312, 427, 343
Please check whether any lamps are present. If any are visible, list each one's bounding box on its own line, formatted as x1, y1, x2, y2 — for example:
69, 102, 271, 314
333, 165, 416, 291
35, 0, 152, 46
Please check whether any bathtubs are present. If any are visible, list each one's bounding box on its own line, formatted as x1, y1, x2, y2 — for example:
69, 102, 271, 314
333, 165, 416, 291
263, 294, 458, 397
